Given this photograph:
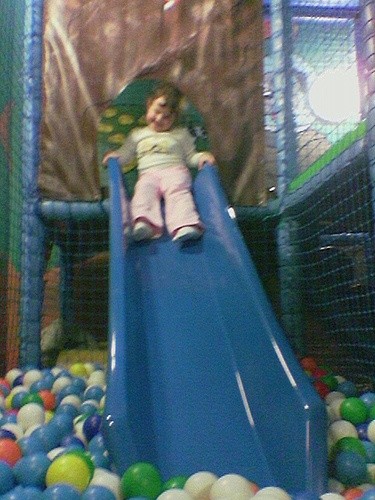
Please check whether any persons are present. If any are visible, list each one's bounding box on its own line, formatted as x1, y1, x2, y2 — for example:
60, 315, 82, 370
41, 250, 108, 365
102, 84, 214, 242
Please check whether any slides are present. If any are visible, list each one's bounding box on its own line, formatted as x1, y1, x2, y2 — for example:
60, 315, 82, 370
103, 153, 329, 496
